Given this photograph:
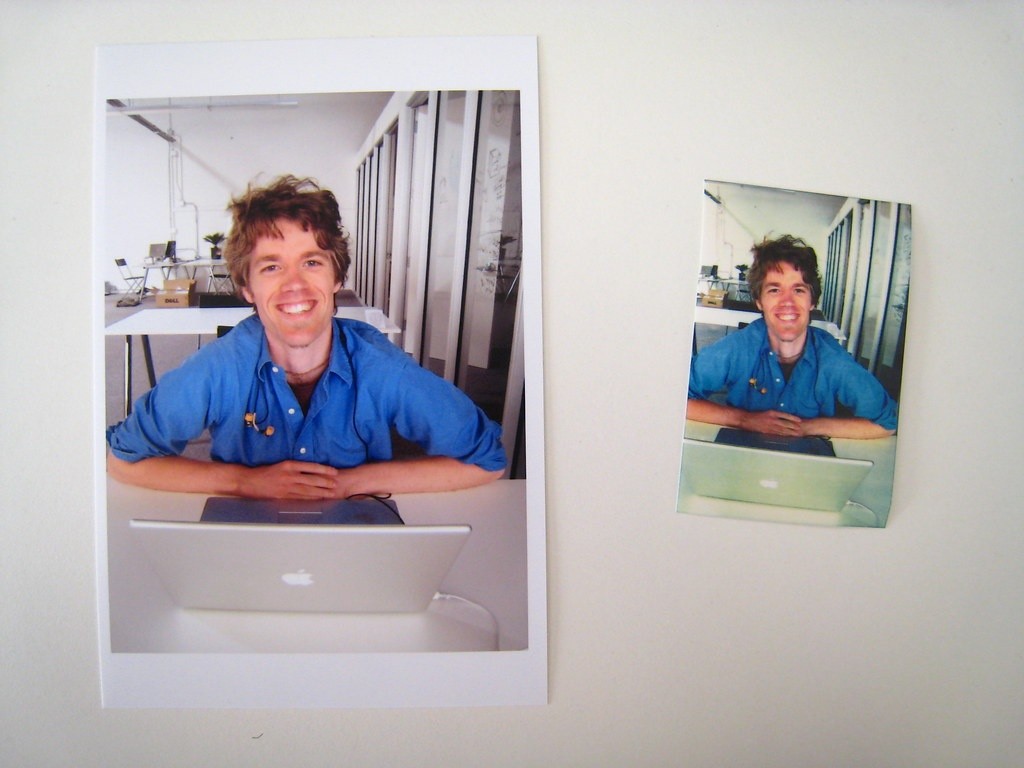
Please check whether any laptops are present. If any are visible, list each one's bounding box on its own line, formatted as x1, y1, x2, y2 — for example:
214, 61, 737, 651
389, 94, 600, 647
682, 427, 875, 512
129, 496, 471, 616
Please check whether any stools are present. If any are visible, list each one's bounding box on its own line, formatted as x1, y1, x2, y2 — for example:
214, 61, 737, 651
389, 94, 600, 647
731, 290, 754, 311
209, 274, 231, 297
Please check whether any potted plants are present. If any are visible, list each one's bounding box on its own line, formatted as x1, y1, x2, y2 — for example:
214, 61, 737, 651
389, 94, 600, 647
735, 264, 751, 281
498, 235, 517, 260
203, 233, 228, 258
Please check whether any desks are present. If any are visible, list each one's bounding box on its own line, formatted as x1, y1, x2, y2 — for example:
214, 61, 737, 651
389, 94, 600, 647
108, 480, 527, 652
105, 307, 401, 419
496, 260, 521, 303
719, 278, 757, 308
693, 306, 847, 355
140, 260, 197, 302
676, 419, 897, 527
181, 258, 235, 297
698, 278, 729, 298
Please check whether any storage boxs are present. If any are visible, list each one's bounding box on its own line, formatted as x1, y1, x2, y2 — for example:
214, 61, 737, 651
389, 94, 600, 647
701, 289, 729, 308
152, 278, 197, 307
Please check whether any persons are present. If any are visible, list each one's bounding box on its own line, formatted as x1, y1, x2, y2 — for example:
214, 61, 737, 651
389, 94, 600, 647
105, 174, 508, 498
686, 234, 897, 439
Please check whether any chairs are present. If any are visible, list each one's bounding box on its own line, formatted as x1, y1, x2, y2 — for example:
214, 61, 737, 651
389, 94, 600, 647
115, 258, 146, 299
